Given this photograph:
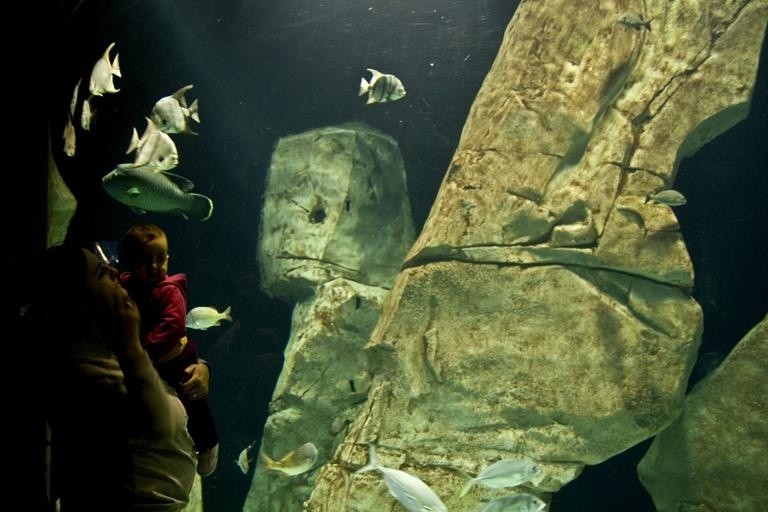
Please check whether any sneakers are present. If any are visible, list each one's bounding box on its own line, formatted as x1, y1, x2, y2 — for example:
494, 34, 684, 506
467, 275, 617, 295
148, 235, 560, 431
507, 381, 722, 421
197, 442, 219, 477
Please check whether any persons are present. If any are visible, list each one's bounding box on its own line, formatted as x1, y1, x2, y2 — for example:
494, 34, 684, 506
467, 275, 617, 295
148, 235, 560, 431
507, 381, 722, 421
104, 221, 219, 477
21, 166, 203, 512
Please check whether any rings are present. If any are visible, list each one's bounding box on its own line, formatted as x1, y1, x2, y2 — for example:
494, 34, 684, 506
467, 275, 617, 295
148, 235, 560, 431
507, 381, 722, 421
192, 392, 197, 399
